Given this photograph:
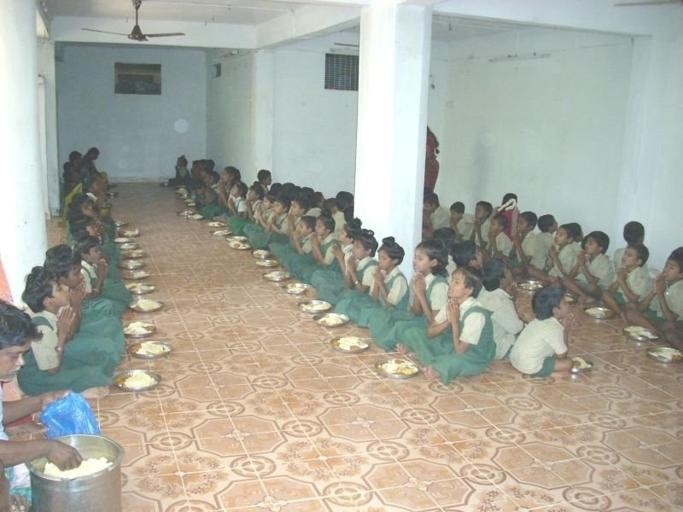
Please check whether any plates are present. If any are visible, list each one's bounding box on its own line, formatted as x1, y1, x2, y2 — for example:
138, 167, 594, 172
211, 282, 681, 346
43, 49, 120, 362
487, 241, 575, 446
124, 299, 163, 313
562, 290, 578, 305
564, 359, 594, 374
208, 228, 232, 236
110, 368, 161, 392
373, 358, 421, 381
121, 320, 156, 338
175, 184, 188, 199
119, 228, 139, 237
583, 304, 617, 320
205, 219, 227, 228
224, 235, 247, 242
281, 281, 310, 296
119, 259, 144, 270
127, 340, 173, 358
176, 208, 196, 216
251, 249, 270, 259
516, 279, 546, 292
254, 259, 279, 268
645, 345, 683, 364
312, 312, 351, 328
183, 199, 197, 207
123, 282, 155, 295
622, 324, 660, 343
119, 269, 150, 280
112, 221, 130, 227
112, 237, 133, 243
120, 250, 145, 258
103, 192, 118, 196
160, 182, 172, 186
185, 214, 204, 220
330, 336, 371, 354
260, 269, 292, 283
227, 241, 252, 250
293, 298, 332, 314
119, 243, 138, 250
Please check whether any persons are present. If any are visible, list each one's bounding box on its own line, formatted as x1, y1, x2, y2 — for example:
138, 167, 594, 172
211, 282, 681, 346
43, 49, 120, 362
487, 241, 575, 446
16, 266, 120, 400
59, 147, 128, 322
42, 243, 129, 358
423, 124, 442, 199
1, 300, 83, 512
392, 192, 683, 386
163, 156, 408, 338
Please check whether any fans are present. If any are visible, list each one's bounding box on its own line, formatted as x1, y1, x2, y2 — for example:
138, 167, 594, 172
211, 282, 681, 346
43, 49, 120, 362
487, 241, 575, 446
79, 1, 186, 43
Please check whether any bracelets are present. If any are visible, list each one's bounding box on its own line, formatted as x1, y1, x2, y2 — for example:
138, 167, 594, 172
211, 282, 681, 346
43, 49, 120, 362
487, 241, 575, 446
55, 346, 65, 353
